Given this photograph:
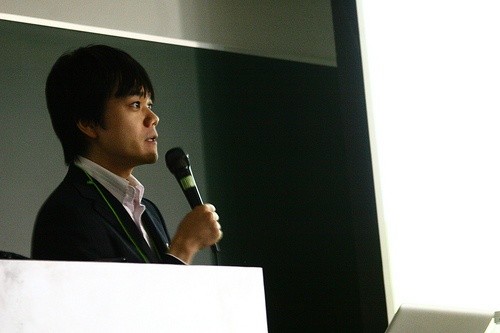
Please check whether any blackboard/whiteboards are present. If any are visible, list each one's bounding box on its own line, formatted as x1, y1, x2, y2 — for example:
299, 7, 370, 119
0, 12, 399, 333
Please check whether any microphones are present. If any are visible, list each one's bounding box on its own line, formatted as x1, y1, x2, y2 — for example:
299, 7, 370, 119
165, 147, 220, 254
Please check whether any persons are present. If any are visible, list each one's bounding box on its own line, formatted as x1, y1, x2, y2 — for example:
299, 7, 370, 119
31, 44, 223, 265
